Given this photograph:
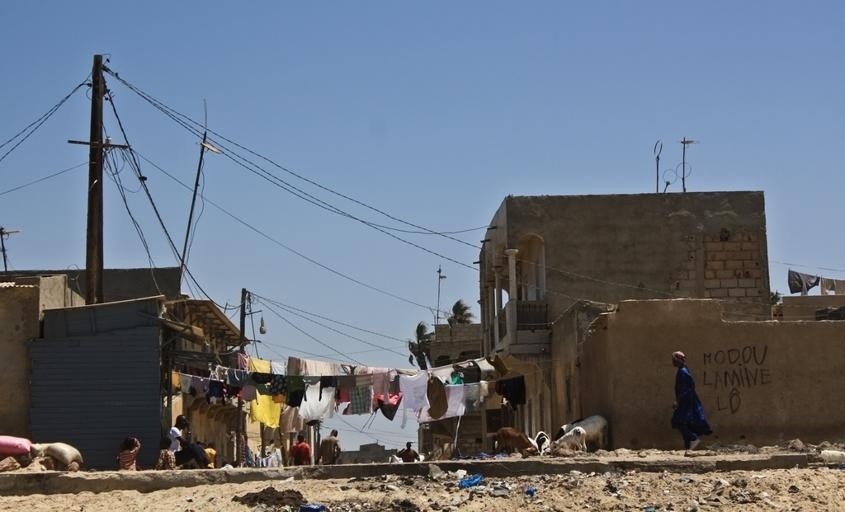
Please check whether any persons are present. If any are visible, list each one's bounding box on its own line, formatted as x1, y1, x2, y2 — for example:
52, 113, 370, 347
167, 415, 210, 469
671, 350, 713, 450
292, 434, 312, 465
315, 429, 341, 464
155, 436, 176, 469
396, 441, 420, 462
117, 437, 141, 470
203, 441, 217, 469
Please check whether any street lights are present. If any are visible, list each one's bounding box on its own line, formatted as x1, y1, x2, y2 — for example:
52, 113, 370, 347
239, 287, 267, 464
436, 263, 448, 323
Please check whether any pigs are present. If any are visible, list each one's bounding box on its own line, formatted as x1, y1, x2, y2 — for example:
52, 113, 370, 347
496, 427, 540, 459
535, 431, 553, 455
553, 414, 609, 448
527, 436, 541, 454
553, 427, 588, 452
